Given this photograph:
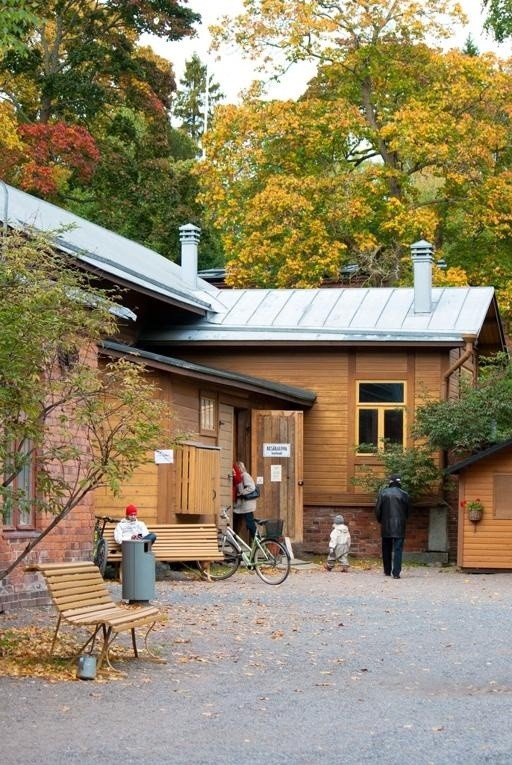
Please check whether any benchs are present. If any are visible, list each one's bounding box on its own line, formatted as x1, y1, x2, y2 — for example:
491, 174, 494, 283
99, 523, 225, 584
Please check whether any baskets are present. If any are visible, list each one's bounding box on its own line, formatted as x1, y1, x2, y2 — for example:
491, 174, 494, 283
469, 510, 481, 520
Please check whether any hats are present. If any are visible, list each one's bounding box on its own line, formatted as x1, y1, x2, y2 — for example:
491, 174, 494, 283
127, 505, 136, 515
390, 474, 401, 484
335, 515, 344, 524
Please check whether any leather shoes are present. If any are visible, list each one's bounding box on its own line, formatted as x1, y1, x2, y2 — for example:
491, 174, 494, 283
385, 573, 400, 579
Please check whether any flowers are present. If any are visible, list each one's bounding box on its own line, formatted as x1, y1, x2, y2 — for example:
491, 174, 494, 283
461, 498, 482, 510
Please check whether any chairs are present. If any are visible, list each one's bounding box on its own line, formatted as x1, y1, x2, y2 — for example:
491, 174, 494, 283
22, 563, 166, 678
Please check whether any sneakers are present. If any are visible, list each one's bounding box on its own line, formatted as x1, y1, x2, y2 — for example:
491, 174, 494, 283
341, 568, 348, 572
324, 564, 332, 571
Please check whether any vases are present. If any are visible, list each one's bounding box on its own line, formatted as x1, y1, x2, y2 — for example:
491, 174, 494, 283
468, 509, 482, 521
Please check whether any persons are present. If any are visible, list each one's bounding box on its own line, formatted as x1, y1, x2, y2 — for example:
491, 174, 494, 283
113, 503, 157, 546
233, 463, 243, 504
374, 475, 412, 580
234, 462, 260, 544
324, 514, 351, 573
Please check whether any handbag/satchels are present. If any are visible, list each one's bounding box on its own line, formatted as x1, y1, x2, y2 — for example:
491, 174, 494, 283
242, 475, 260, 500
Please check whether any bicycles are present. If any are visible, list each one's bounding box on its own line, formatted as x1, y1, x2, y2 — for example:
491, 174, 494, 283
93, 515, 120, 579
197, 505, 291, 585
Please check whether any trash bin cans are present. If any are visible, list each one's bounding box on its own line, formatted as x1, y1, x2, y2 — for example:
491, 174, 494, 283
122, 540, 156, 605
